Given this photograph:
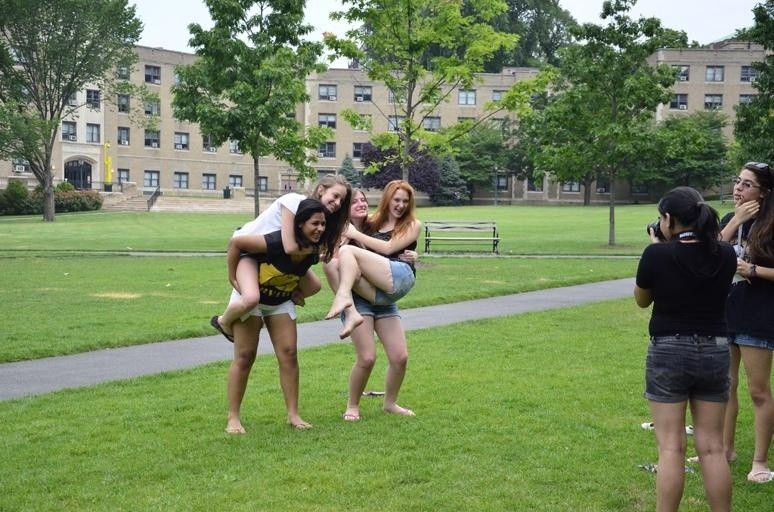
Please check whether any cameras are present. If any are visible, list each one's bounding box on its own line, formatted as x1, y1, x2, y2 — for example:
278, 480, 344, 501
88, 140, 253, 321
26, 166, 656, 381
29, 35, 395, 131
646, 218, 665, 240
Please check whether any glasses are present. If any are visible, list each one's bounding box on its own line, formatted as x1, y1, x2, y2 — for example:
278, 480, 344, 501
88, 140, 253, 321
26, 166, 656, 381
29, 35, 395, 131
732, 178, 771, 192
746, 162, 770, 170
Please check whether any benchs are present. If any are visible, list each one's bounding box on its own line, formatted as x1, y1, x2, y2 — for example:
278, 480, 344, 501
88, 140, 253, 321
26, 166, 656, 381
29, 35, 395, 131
422, 219, 501, 254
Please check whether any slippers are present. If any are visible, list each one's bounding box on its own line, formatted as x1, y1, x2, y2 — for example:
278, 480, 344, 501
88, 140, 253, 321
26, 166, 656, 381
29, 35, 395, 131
749, 472, 774, 483
225, 427, 244, 433
211, 316, 235, 342
295, 423, 310, 427
381, 407, 409, 415
343, 414, 360, 420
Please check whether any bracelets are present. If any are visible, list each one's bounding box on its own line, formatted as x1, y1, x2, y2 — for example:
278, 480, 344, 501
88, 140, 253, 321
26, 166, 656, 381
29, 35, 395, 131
751, 263, 758, 277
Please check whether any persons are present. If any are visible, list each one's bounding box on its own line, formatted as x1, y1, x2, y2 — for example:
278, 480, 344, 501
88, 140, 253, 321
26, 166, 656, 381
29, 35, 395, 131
688, 160, 774, 485
323, 180, 423, 339
225, 199, 329, 435
322, 188, 416, 421
209, 173, 353, 341
632, 185, 735, 511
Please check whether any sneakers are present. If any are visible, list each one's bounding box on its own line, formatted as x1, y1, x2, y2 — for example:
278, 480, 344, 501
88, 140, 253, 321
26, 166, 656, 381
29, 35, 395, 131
686, 425, 694, 434
642, 422, 654, 430
